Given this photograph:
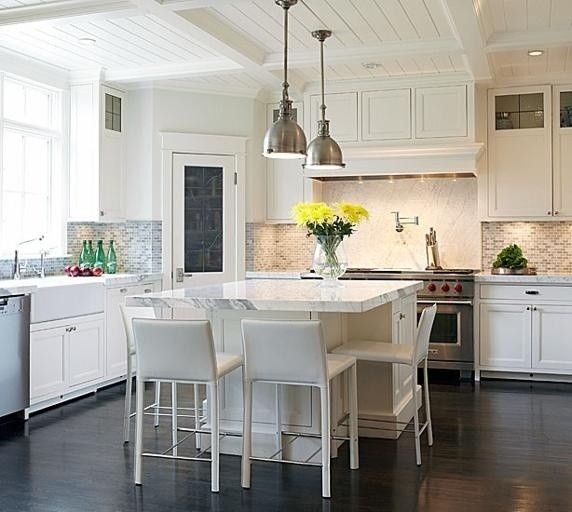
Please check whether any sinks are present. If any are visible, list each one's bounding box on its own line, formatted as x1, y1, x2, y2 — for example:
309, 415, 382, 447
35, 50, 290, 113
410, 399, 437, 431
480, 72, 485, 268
7, 281, 105, 323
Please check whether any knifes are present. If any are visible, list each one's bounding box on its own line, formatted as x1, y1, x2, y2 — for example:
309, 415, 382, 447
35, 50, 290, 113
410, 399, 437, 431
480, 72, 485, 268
425, 226, 438, 247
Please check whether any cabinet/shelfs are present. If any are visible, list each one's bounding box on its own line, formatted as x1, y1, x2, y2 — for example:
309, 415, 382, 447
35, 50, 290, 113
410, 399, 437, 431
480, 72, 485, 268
488, 84, 572, 218
29, 312, 104, 405
106, 282, 161, 383
474, 285, 572, 384
307, 78, 476, 144
350, 293, 417, 411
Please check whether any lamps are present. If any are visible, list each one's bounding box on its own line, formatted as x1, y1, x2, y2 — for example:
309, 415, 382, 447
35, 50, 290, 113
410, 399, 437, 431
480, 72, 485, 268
305, 30, 346, 170
262, 0, 307, 159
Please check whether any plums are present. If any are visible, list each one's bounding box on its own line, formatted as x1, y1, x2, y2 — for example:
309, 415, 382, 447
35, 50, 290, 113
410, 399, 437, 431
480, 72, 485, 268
65, 265, 102, 277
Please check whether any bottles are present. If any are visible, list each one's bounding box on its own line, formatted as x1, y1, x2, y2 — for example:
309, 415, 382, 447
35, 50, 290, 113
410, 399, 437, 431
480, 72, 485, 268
79, 239, 116, 274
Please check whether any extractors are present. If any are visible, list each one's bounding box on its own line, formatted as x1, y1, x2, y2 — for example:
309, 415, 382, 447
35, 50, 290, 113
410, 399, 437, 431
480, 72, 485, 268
305, 146, 479, 184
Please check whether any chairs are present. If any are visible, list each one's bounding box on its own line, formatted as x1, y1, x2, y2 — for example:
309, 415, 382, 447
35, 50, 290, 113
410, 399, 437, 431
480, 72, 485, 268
240, 318, 360, 497
119, 304, 204, 451
132, 317, 245, 493
329, 303, 438, 466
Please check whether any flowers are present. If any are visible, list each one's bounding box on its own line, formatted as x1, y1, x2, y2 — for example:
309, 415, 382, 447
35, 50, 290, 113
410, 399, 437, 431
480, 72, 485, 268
292, 202, 371, 277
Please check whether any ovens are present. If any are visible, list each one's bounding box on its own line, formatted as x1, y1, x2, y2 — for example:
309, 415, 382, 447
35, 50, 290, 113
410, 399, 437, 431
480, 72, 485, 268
418, 295, 474, 379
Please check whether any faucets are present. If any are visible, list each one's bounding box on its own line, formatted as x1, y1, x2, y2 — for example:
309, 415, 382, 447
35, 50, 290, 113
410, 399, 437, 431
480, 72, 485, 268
391, 209, 420, 229
13, 235, 43, 279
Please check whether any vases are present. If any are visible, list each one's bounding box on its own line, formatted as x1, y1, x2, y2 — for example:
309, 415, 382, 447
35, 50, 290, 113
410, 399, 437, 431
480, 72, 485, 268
313, 235, 349, 289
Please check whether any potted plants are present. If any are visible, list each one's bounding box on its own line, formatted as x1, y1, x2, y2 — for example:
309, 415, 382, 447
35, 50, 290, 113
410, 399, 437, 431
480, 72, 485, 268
491, 243, 528, 274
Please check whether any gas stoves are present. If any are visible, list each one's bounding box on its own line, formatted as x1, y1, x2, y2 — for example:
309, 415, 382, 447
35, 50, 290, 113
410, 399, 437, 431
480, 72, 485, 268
300, 267, 476, 297
